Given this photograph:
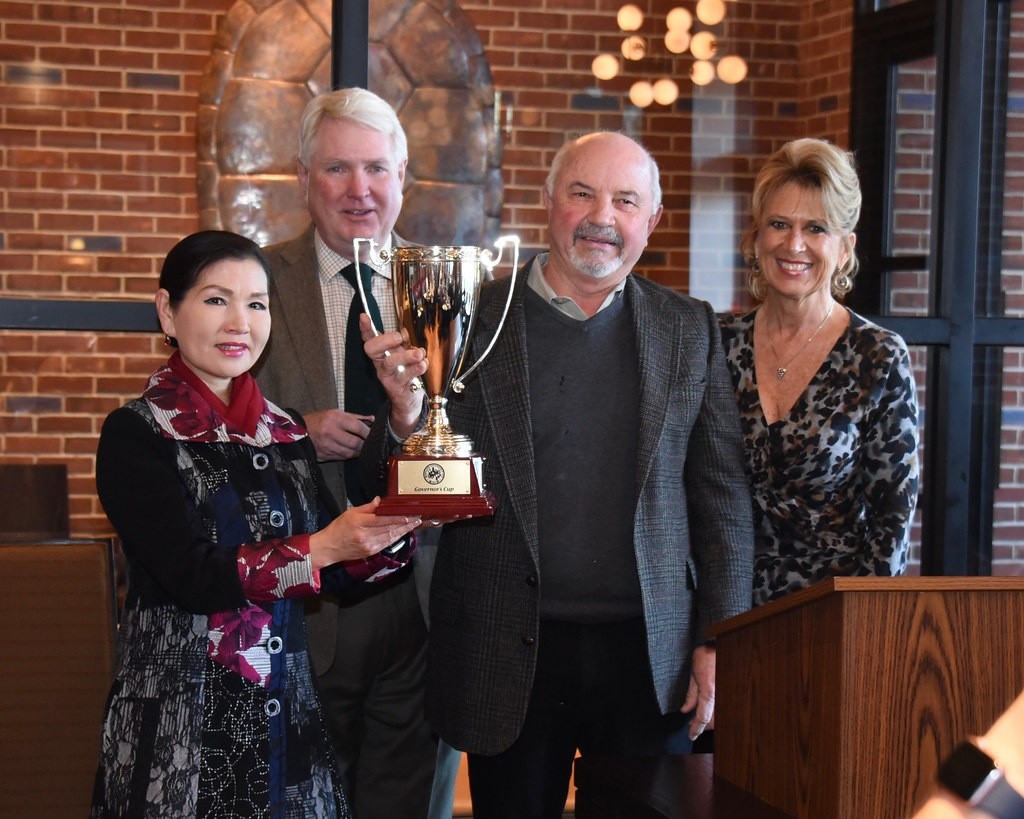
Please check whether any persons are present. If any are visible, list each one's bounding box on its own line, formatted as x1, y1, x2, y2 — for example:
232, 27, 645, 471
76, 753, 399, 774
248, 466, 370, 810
93, 230, 420, 819
351, 130, 754, 819
914, 689, 1024, 819
252, 86, 461, 819
720, 136, 922, 604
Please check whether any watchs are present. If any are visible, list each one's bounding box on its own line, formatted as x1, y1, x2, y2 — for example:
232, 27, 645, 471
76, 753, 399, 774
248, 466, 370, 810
936, 742, 1024, 819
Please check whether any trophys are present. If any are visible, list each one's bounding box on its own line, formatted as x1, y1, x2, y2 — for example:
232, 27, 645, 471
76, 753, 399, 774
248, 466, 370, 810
353, 234, 519, 518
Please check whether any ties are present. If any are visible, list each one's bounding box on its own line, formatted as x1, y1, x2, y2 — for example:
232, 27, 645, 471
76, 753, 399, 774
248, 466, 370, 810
340, 263, 392, 509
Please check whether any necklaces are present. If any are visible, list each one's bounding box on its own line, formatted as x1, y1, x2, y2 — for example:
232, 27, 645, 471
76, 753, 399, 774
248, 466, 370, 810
767, 303, 834, 379
